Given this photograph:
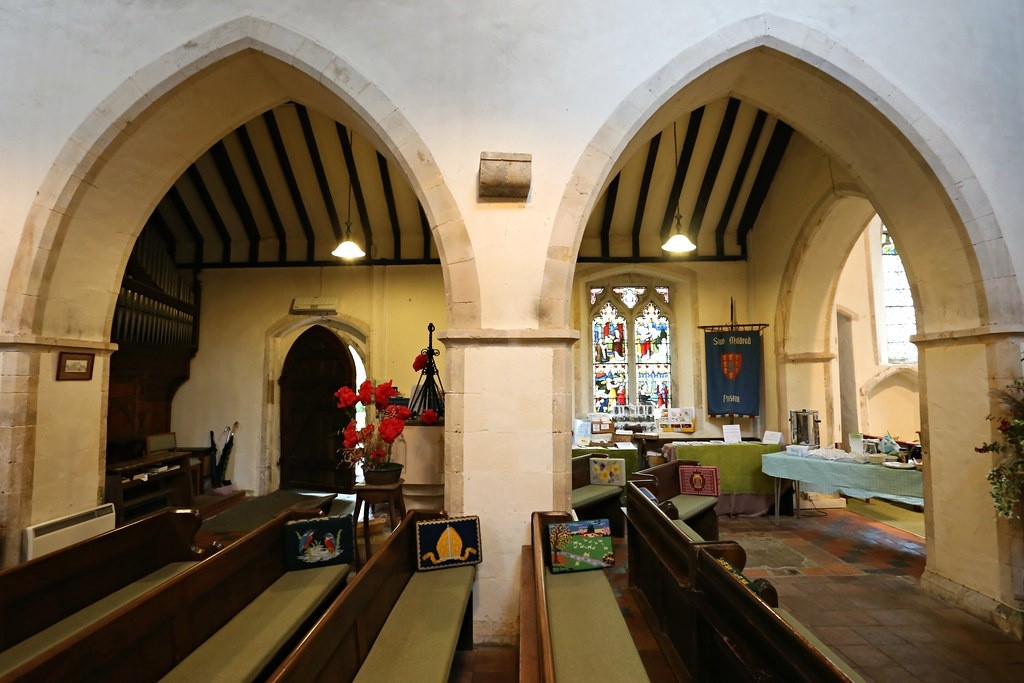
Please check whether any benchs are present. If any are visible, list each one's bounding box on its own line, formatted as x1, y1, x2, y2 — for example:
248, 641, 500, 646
0, 455, 866, 683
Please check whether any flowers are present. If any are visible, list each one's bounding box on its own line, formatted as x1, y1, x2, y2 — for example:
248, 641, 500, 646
335, 354, 438, 476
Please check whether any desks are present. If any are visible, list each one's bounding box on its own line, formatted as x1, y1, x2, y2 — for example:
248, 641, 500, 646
574, 447, 642, 500
761, 451, 923, 519
667, 440, 786, 512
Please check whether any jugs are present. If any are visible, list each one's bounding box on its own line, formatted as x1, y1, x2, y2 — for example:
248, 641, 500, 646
849, 432, 863, 454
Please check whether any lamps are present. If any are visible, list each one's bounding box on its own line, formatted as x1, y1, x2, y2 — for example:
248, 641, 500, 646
332, 131, 366, 259
660, 122, 697, 253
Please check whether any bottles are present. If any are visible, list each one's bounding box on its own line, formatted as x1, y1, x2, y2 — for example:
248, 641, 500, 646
898, 448, 908, 463
865, 443, 876, 454
911, 445, 922, 459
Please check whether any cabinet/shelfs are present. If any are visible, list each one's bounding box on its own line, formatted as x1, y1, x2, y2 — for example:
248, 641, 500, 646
107, 451, 197, 517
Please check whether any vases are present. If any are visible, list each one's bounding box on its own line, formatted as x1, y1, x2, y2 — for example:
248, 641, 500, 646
361, 463, 404, 484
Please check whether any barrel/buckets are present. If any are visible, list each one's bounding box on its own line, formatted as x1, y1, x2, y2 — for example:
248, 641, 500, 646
788, 408, 821, 446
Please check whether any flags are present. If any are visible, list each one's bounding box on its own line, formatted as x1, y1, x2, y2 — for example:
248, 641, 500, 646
703, 330, 760, 417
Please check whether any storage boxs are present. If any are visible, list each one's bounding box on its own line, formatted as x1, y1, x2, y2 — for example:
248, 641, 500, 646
785, 445, 816, 457
592, 422, 615, 433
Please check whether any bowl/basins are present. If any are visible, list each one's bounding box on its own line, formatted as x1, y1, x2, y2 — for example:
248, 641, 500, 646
870, 454, 898, 463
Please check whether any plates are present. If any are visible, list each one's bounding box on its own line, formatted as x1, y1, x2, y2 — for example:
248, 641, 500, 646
882, 462, 915, 468
